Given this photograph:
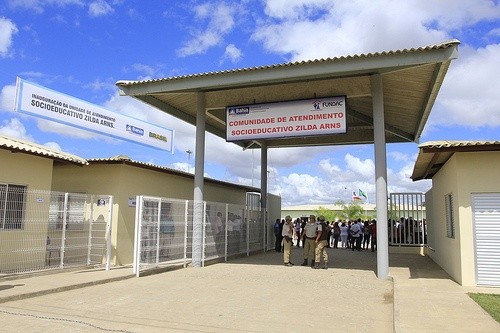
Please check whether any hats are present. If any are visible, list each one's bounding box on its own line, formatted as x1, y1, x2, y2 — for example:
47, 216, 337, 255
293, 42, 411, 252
285, 216, 291, 221
319, 215, 325, 220
309, 215, 315, 219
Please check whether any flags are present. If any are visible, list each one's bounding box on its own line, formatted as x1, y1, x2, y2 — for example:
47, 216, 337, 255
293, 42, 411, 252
359, 189, 368, 200
353, 192, 361, 200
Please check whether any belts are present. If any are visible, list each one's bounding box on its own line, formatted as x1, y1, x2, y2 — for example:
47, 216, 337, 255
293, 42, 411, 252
305, 236, 317, 239
283, 236, 292, 240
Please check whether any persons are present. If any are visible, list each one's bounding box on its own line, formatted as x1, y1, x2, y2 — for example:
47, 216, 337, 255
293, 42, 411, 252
273, 214, 427, 269
141, 210, 242, 259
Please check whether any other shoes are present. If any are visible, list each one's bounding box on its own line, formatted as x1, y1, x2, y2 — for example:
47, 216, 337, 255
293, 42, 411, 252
285, 263, 292, 266
289, 261, 294, 265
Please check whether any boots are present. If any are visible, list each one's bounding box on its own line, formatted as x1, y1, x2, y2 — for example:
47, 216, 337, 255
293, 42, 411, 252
312, 262, 320, 269
310, 260, 315, 266
302, 259, 307, 266
322, 261, 328, 269
